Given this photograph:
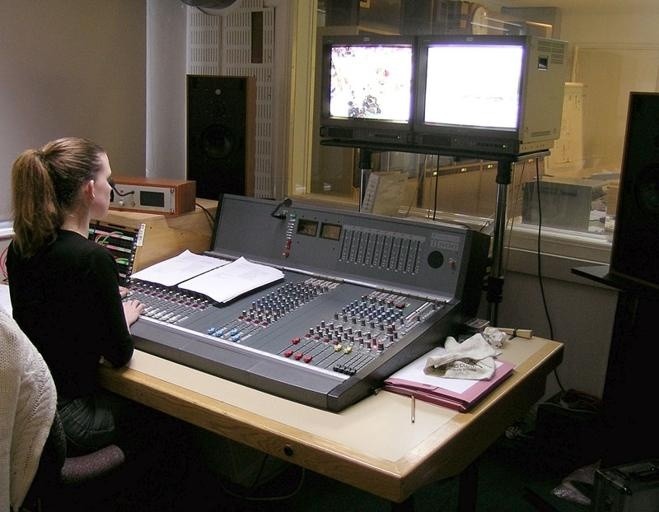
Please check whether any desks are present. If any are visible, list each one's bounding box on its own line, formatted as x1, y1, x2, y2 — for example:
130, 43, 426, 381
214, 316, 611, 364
91, 333, 565, 512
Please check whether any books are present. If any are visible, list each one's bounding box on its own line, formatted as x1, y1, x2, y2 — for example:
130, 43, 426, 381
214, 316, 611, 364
384, 345, 516, 407
385, 370, 514, 413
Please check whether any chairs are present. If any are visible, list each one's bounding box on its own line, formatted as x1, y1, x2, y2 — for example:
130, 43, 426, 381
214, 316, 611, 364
1, 305, 127, 512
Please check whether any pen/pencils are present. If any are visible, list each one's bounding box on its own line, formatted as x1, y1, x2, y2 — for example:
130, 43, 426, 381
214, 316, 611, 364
411, 395, 416, 424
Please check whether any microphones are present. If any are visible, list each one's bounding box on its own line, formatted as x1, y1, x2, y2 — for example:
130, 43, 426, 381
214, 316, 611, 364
270, 199, 292, 219
108, 177, 134, 196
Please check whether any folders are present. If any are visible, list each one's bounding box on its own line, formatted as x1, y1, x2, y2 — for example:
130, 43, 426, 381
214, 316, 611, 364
361, 171, 409, 213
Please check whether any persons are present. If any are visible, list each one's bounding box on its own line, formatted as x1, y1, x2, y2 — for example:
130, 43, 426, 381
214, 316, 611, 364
8, 136, 150, 456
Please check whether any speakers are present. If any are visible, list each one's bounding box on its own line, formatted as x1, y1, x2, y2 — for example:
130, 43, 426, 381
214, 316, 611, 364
186, 74, 257, 201
610, 93, 659, 292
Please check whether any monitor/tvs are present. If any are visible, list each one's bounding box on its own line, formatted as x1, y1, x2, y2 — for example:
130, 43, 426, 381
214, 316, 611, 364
415, 35, 568, 156
320, 36, 419, 147
